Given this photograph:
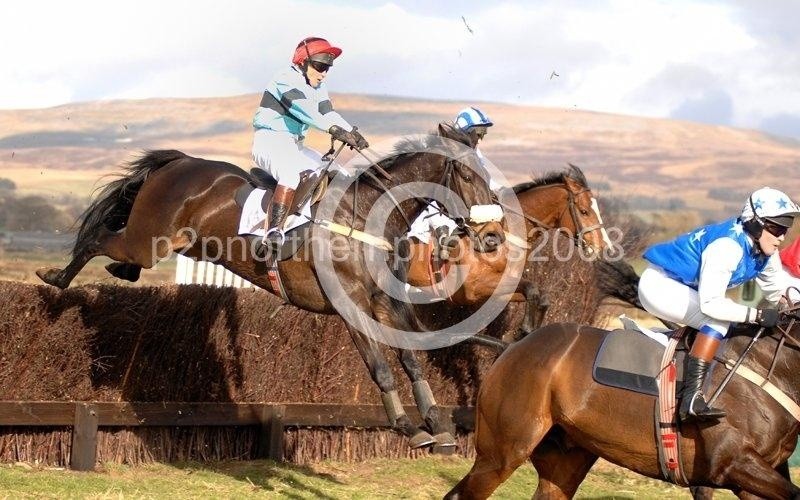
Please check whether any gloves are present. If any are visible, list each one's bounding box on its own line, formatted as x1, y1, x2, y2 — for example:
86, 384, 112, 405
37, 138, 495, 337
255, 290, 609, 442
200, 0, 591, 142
329, 125, 370, 150
755, 308, 779, 328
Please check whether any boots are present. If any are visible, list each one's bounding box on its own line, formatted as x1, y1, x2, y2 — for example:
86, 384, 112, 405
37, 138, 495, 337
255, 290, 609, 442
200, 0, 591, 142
266, 202, 289, 249
434, 225, 459, 247
679, 355, 726, 419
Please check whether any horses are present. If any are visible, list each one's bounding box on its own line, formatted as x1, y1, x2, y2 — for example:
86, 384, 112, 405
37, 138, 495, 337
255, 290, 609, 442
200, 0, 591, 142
406, 162, 615, 338
33, 120, 507, 453
443, 303, 800, 500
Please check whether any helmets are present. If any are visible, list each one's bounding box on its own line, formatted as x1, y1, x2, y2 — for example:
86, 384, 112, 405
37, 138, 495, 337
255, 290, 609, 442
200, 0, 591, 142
453, 106, 494, 131
741, 187, 800, 228
292, 36, 342, 65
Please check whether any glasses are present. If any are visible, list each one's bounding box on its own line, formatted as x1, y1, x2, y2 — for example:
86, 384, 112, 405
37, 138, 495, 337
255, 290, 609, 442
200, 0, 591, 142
305, 45, 330, 73
750, 196, 787, 237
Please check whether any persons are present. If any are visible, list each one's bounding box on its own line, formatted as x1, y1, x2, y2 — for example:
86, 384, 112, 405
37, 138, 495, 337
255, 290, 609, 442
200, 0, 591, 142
250, 37, 369, 246
407, 106, 509, 249
637, 187, 800, 423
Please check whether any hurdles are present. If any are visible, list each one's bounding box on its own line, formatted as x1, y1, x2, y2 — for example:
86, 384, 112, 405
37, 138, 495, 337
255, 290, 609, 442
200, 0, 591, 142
1, 229, 640, 470
